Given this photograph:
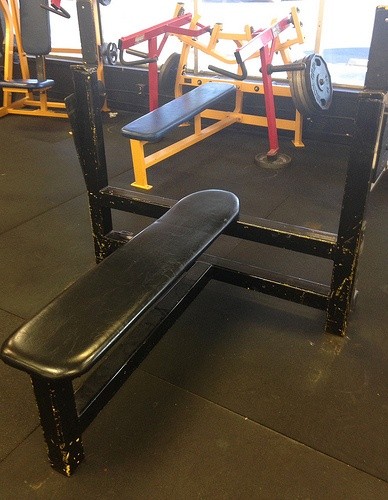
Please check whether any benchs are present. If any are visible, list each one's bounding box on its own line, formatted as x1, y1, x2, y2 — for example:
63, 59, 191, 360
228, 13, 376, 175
0, 189, 239, 477
121, 82, 236, 190
